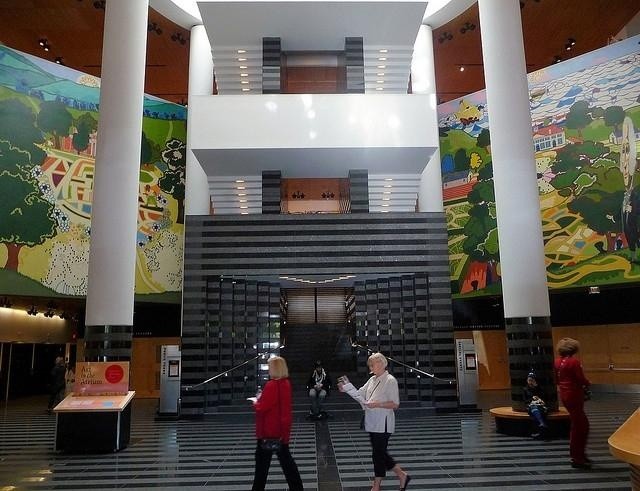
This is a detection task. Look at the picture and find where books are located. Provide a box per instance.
[343,383,365,404]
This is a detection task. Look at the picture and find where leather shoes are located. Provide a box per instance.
[400,474,410,491]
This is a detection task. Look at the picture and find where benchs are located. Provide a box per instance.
[488,403,574,441]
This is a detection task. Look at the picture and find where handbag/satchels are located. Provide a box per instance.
[360,410,365,429]
[530,396,545,406]
[261,438,281,452]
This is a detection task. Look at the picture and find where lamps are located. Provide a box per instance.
[551,38,577,66]
[291,190,305,200]
[0,296,73,321]
[93,0,187,45]
[321,192,336,199]
[38,37,63,66]
[437,21,475,43]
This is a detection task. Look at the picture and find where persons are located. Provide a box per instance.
[306,359,329,418]
[523,371,550,438]
[337,352,411,491]
[555,337,594,468]
[45,356,66,415]
[246,356,304,490]
